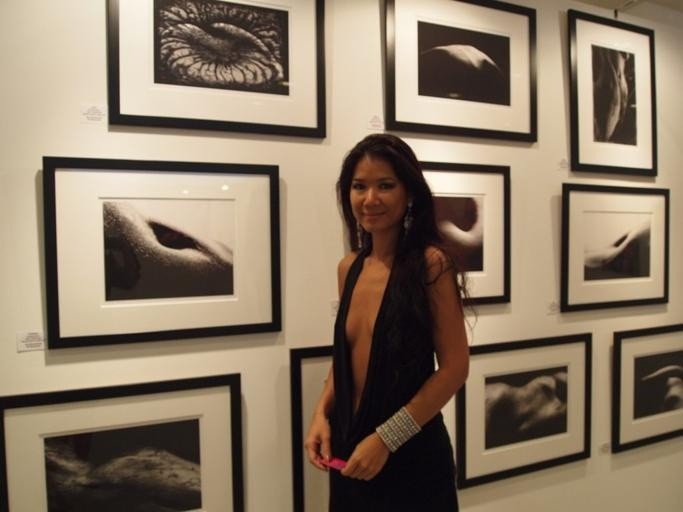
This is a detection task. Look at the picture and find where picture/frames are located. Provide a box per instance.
[105,0,326,139]
[612,323,683,454]
[561,183,669,312]
[419,161,511,304]
[0,373,244,511]
[567,8,658,176]
[290,345,334,512]
[456,333,592,489]
[43,155,281,348]
[385,1,537,142]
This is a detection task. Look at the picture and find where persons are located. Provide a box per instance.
[304,135,478,512]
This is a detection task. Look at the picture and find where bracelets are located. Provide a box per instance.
[374,406,420,454]
